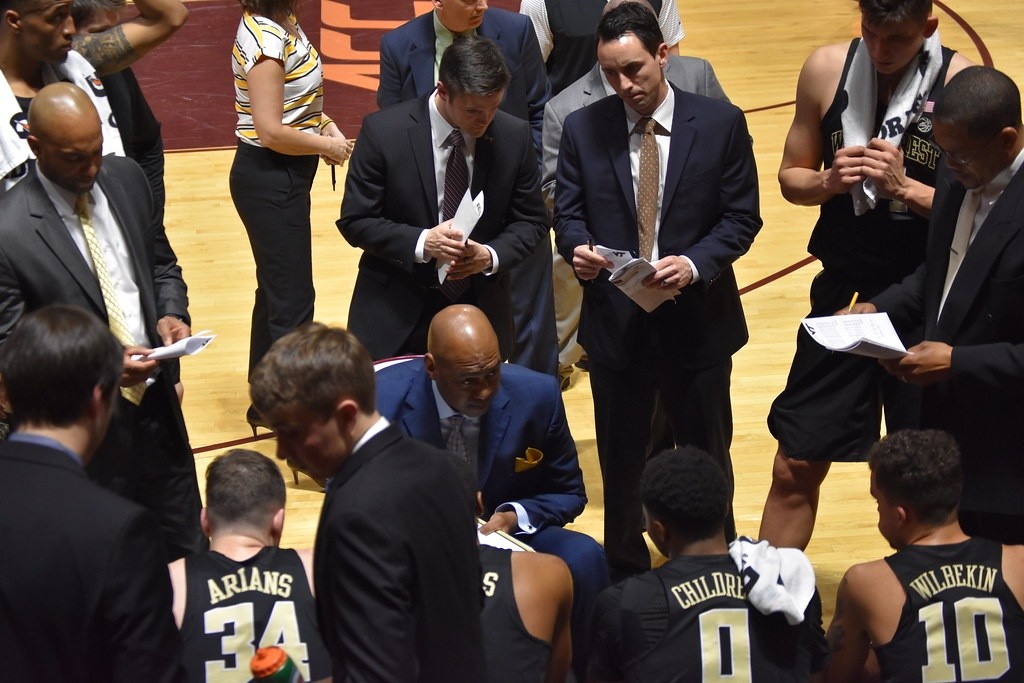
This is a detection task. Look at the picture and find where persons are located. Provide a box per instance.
[0,0,1024,682]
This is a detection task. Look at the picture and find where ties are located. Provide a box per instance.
[447,416,472,467]
[440,132,472,303]
[635,118,657,263]
[937,183,985,324]
[452,32,463,43]
[76,189,147,406]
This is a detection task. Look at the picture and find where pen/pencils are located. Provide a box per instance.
[588,234,594,284]
[330,164,336,191]
[831,291,859,355]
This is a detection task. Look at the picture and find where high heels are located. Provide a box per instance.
[246,402,278,438]
[287,455,326,488]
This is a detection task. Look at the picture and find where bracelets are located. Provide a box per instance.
[321,119,334,130]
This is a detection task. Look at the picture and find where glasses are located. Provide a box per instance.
[927,125,1001,169]
